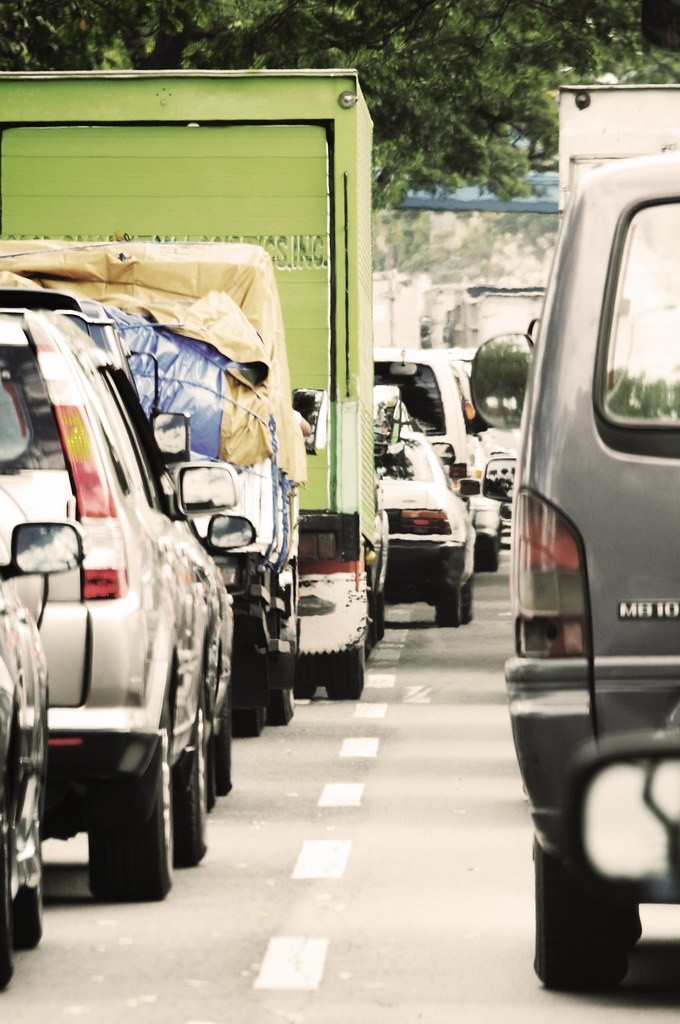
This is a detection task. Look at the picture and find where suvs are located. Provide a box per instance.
[1,307,210,902]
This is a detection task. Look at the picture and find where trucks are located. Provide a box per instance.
[0,68,379,702]
[558,85,680,387]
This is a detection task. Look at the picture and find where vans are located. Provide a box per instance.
[468,148,680,993]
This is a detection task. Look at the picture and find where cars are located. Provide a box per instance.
[1,285,256,811]
[374,347,522,628]
[1,582,49,989]
